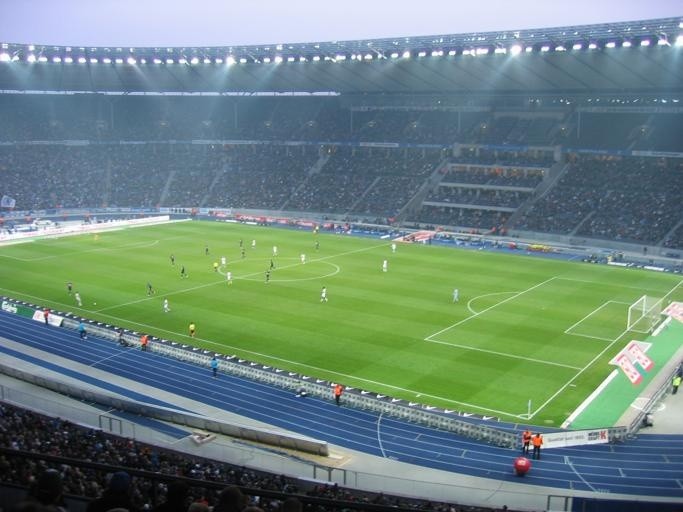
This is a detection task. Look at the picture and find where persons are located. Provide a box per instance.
[335,384,342,404]
[78,322,87,340]
[226,271,232,286]
[521,429,532,455]
[163,298,170,313]
[180,264,187,279]
[451,287,458,303]
[114,329,122,347]
[1,403,350,511]
[382,260,387,272]
[269,259,274,270]
[531,432,543,460]
[319,286,328,303]
[146,282,155,296]
[210,352,218,380]
[345,487,485,512]
[67,282,72,295]
[140,334,148,351]
[265,270,270,284]
[170,254,175,266]
[189,322,195,337]
[0,109,683,264]
[43,309,50,324]
[220,256,225,267]
[213,262,218,272]
[75,291,82,306]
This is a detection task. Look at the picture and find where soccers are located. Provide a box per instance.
[94,303,96,305]
